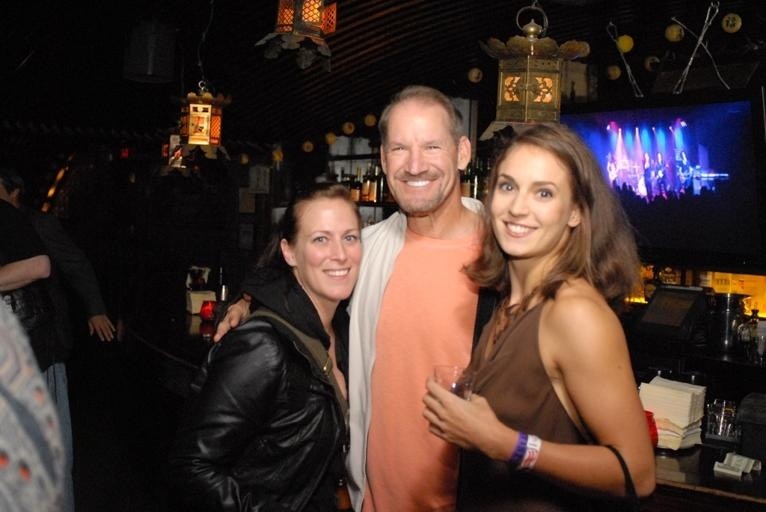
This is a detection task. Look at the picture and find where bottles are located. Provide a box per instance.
[736,308,766,368]
[214,285,230,329]
[460,156,492,201]
[337,161,388,204]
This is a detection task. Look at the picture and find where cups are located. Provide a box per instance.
[432,364,477,401]
[200,301,218,321]
[704,398,738,437]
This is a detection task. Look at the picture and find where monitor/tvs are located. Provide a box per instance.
[561,85,766,277]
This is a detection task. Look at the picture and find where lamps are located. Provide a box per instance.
[173,48,226,152]
[274,0,341,53]
[482,1,592,132]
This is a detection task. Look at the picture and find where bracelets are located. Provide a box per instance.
[508,432,541,477]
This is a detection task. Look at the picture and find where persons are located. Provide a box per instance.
[641,151,654,202]
[418,118,659,512]
[0,164,116,512]
[181,179,367,511]
[654,150,669,196]
[210,81,510,512]
[675,148,694,196]
